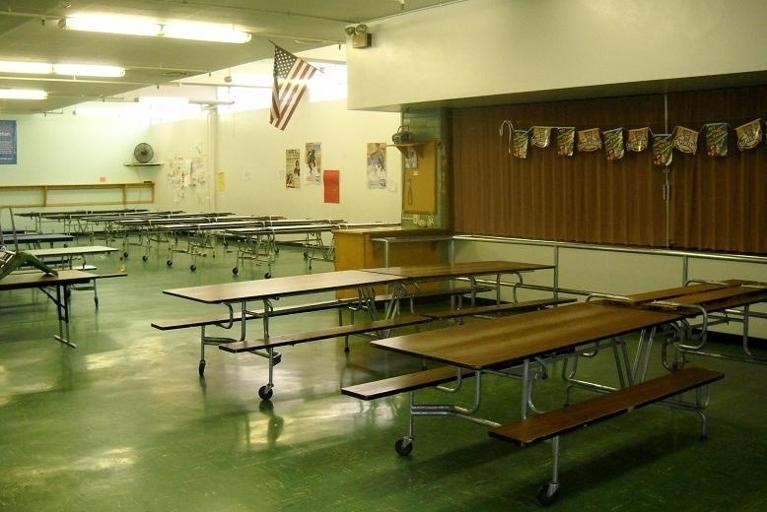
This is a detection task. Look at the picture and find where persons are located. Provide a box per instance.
[304,150,320,178]
[293,156,300,176]
[287,171,293,187]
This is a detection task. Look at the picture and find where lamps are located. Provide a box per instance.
[52,14,251,45]
[345,22,372,49]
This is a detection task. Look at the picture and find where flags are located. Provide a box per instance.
[265,43,318,131]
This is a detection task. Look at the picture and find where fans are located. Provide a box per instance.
[133,142,154,163]
